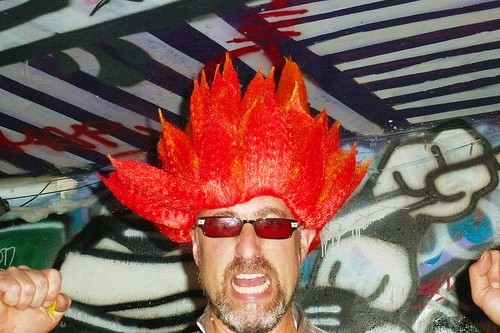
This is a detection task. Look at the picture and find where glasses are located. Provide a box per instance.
[196,216,300,239]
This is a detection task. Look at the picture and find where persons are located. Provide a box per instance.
[0,186,500,333]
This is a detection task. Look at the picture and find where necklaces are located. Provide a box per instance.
[210,307,217,333]
